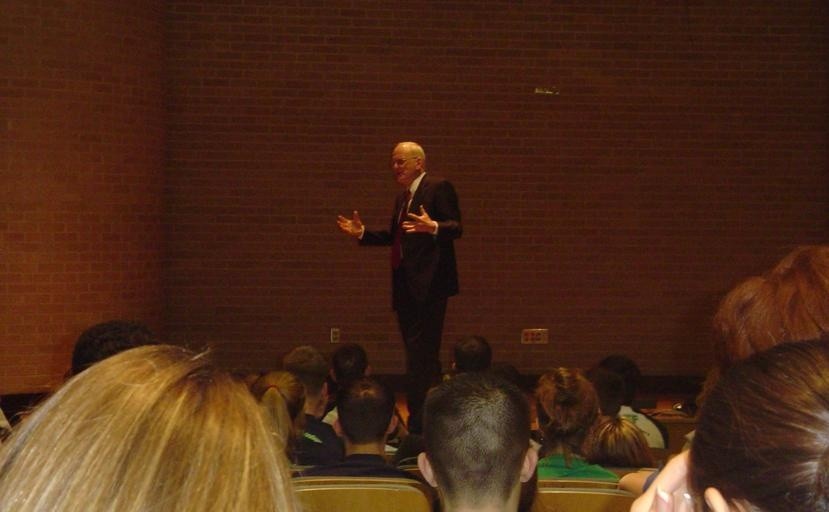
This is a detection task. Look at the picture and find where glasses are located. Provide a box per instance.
[391,156,417,167]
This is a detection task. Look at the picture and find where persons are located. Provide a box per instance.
[0,344,303,512]
[62,321,158,382]
[233,336,670,512]
[629,339,829,512]
[695,245,829,423]
[337,140,464,434]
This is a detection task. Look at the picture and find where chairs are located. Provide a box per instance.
[281,406,703,510]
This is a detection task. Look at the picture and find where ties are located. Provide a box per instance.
[391,189,412,270]
